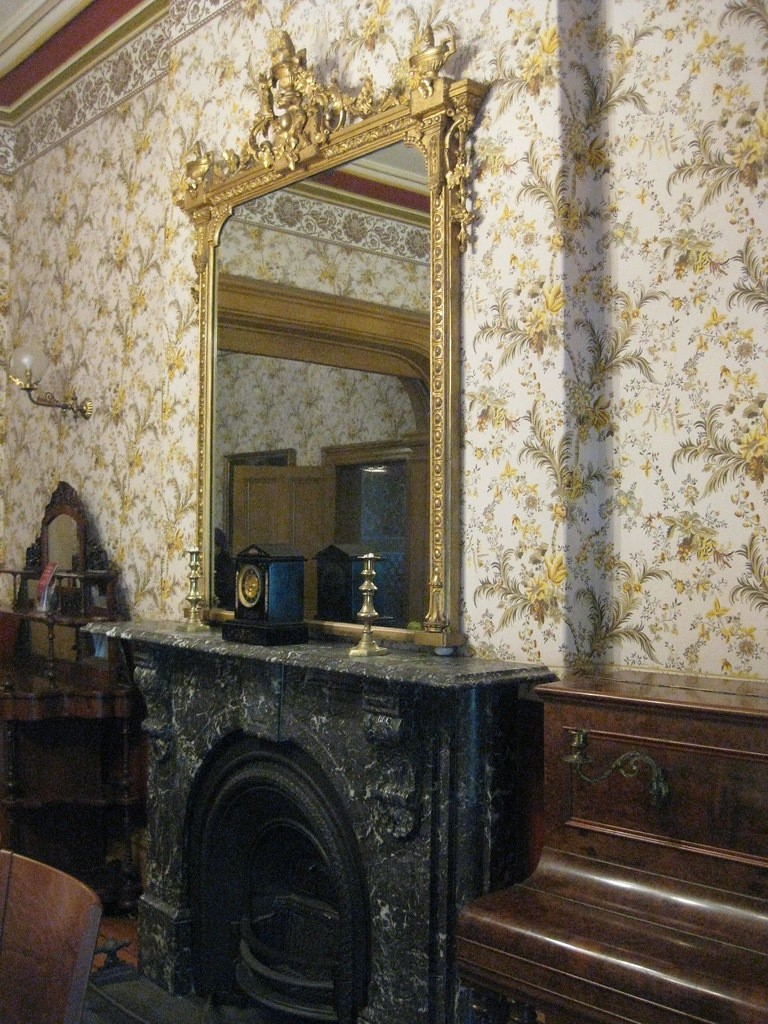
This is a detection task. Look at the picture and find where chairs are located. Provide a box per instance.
[0,849,104,1024]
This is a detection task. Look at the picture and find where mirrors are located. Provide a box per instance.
[169,25,497,652]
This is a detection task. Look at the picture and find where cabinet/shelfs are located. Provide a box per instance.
[2,483,141,918]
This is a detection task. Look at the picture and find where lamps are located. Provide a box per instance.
[6,346,94,420]
